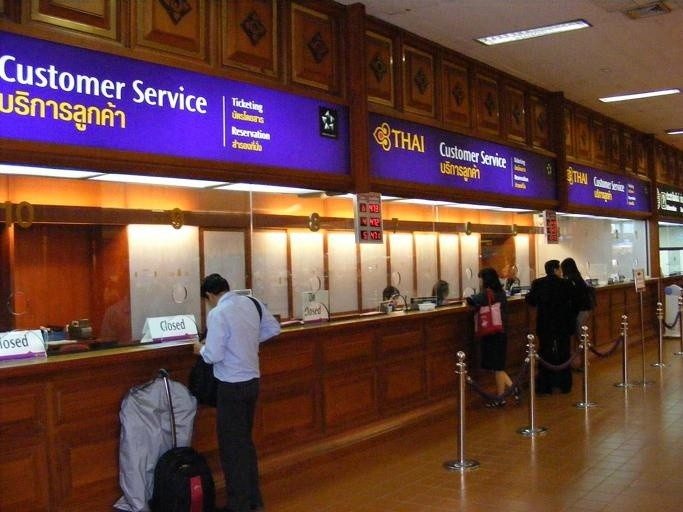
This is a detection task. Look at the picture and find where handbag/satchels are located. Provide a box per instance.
[575,285,597,311]
[471,288,504,337]
[190,359,218,406]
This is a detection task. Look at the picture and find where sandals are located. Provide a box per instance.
[484,397,507,408]
[510,381,521,408]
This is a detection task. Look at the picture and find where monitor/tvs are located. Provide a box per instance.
[410,296,438,309]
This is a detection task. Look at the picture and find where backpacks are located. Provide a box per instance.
[148,446,216,512]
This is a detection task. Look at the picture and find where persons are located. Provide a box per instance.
[504,276,520,292]
[464,266,523,409]
[560,257,594,372]
[524,259,581,395]
[430,279,450,300]
[191,270,283,511]
[381,286,400,302]
